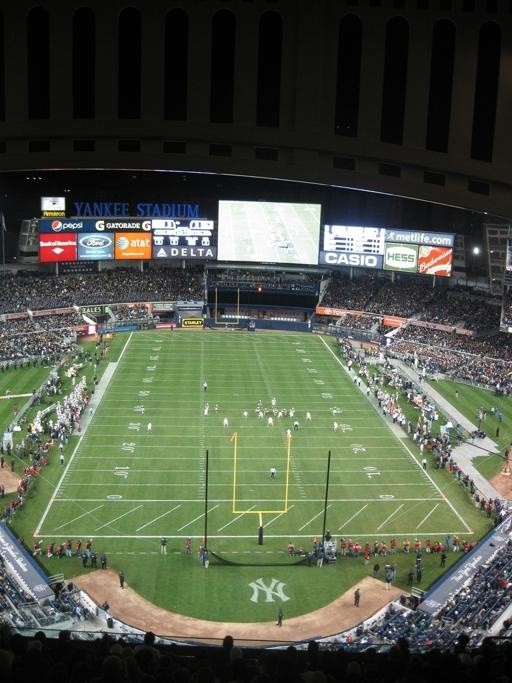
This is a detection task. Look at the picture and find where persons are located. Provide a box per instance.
[0,262,512,683]
